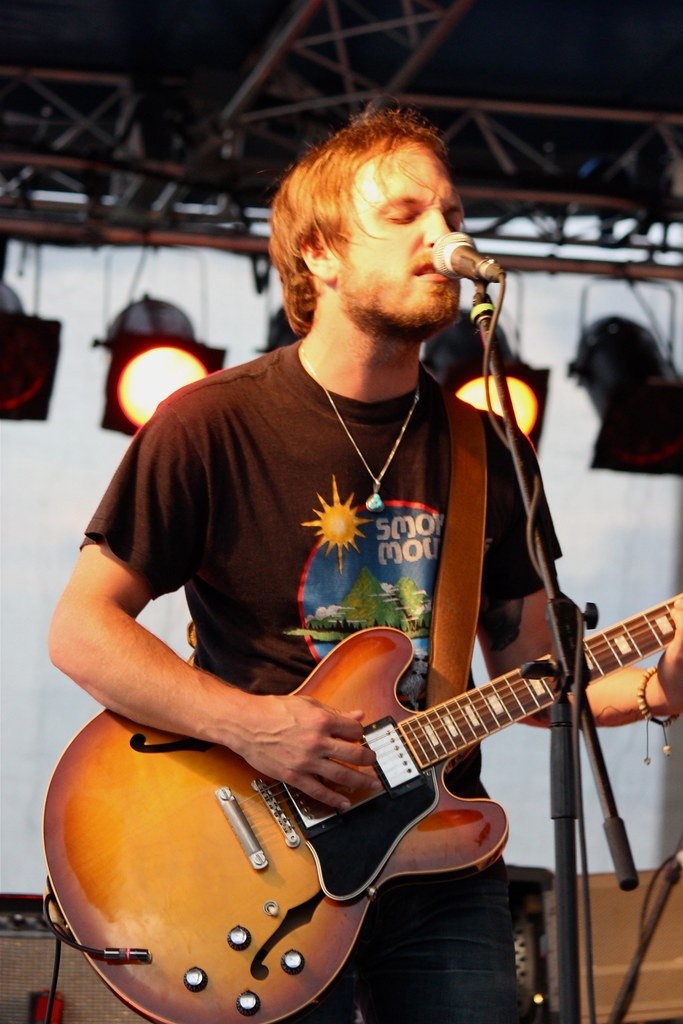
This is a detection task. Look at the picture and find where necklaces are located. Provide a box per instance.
[298,346,422,512]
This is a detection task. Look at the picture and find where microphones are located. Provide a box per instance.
[41,897,151,963]
[432,232,507,284]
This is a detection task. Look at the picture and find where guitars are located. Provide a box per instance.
[39,590,683,1022]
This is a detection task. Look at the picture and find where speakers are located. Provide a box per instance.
[0,893,152,1024]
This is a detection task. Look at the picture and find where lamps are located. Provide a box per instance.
[567,313,683,480]
[424,305,551,451]
[0,264,63,425]
[97,295,228,442]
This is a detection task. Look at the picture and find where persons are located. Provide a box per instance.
[46,115,683,1023]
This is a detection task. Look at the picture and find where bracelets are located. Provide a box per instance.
[636,665,680,765]
